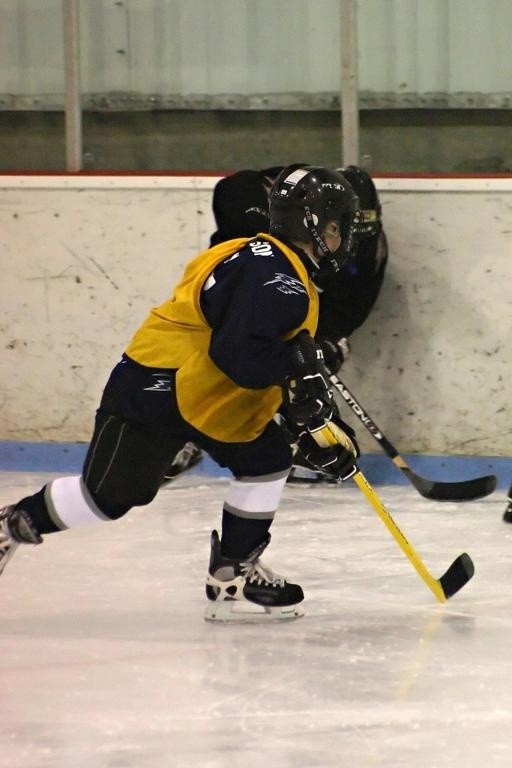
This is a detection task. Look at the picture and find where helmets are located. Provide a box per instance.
[267,164,363,271]
[337,165,382,240]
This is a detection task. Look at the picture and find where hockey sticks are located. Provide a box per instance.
[285,375,476,604]
[320,360,497,502]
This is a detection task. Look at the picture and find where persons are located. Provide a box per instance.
[211,151,388,487]
[0,159,364,610]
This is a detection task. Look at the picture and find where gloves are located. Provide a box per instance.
[288,420,361,479]
[275,365,335,423]
[320,338,350,371]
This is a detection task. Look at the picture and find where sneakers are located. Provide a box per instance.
[165,443,203,477]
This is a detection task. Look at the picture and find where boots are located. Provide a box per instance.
[207,531,303,605]
[0,505,43,574]
